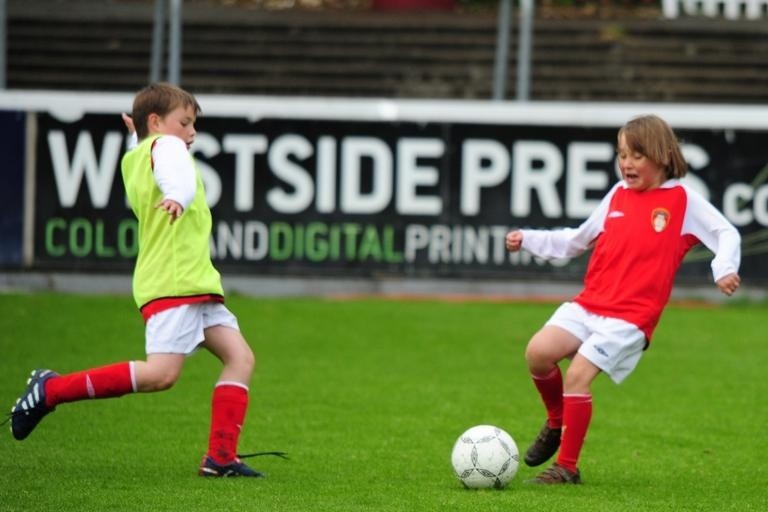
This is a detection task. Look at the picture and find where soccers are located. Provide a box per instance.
[452,424,519,490]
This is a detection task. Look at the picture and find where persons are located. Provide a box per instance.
[9,81,265,478]
[500,114,744,485]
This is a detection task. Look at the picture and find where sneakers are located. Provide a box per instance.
[2,368,61,440]
[199,453,261,479]
[536,465,581,486]
[523,420,560,466]
